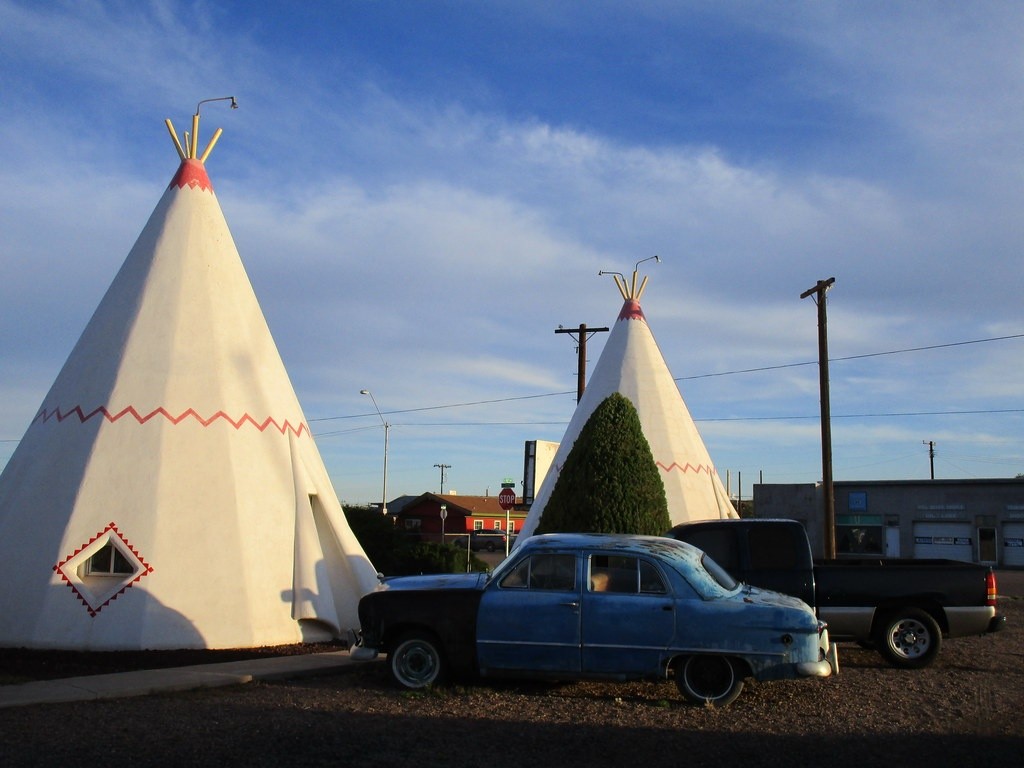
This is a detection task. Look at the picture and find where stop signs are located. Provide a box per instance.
[498,488,516,510]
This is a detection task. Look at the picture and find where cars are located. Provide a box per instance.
[348,533,840,709]
[454,529,518,552]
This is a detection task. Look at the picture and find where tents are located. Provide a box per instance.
[0,157,382,652]
[509,299,741,554]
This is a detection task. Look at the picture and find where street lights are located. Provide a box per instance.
[360,389,392,515]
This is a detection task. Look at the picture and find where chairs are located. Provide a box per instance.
[534,571,573,591]
[590,570,625,592]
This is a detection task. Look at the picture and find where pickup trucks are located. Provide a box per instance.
[659,518,1006,670]
